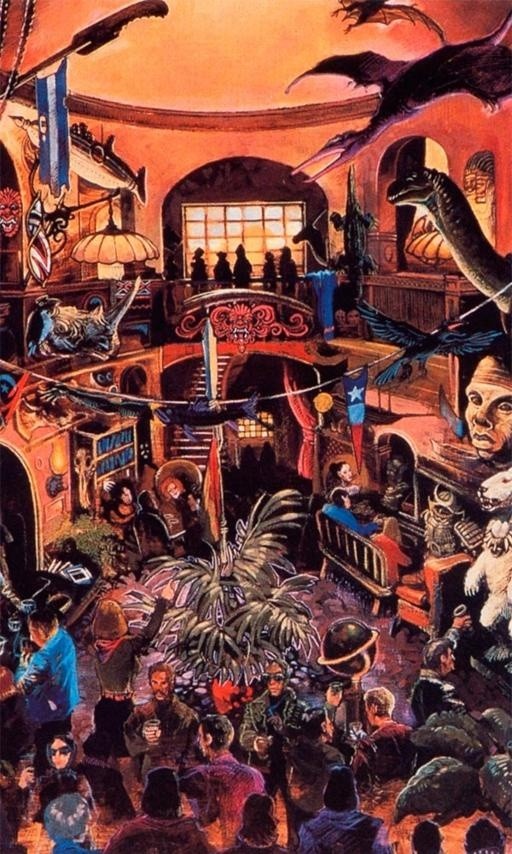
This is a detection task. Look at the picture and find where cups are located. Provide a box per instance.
[145,718,162,747]
[349,720,363,742]
